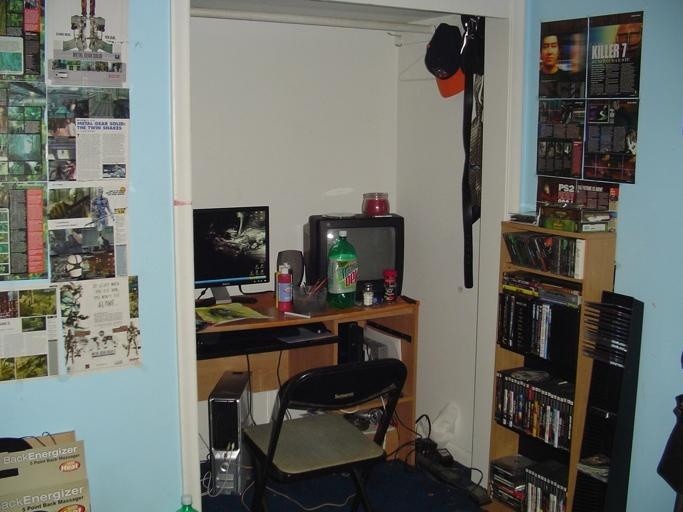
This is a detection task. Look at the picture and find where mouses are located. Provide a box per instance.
[307,319,325,335]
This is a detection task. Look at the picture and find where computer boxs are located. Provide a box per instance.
[209,370,250,494]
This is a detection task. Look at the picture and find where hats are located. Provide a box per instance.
[424,22,464,99]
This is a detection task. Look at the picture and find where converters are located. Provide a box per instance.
[437,448,455,465]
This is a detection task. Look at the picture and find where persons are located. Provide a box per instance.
[539,33,569,98]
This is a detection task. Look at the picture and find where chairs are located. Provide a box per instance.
[243,359,409,512]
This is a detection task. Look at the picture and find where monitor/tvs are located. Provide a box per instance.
[309,212,405,296]
[195,206,269,305]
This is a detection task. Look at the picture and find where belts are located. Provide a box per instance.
[464,17,473,288]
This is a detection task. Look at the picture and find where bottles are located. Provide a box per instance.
[362,283,374,308]
[362,192,391,217]
[325,230,360,310]
[383,268,399,304]
[277,269,294,312]
[275,265,283,309]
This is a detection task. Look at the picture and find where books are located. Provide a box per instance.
[576,453,610,483]
[503,231,585,280]
[498,290,552,360]
[494,365,573,453]
[490,455,539,512]
[525,463,567,512]
[536,202,611,233]
[502,272,582,310]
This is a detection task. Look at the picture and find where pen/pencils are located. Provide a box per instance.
[303,276,330,295]
[285,311,311,318]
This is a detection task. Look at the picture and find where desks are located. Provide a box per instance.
[196,287,421,467]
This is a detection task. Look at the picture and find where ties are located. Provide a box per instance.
[468,73,484,222]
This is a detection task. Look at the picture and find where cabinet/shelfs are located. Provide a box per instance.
[336,309,417,468]
[487,221,615,511]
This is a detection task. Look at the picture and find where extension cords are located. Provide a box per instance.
[414,439,470,483]
